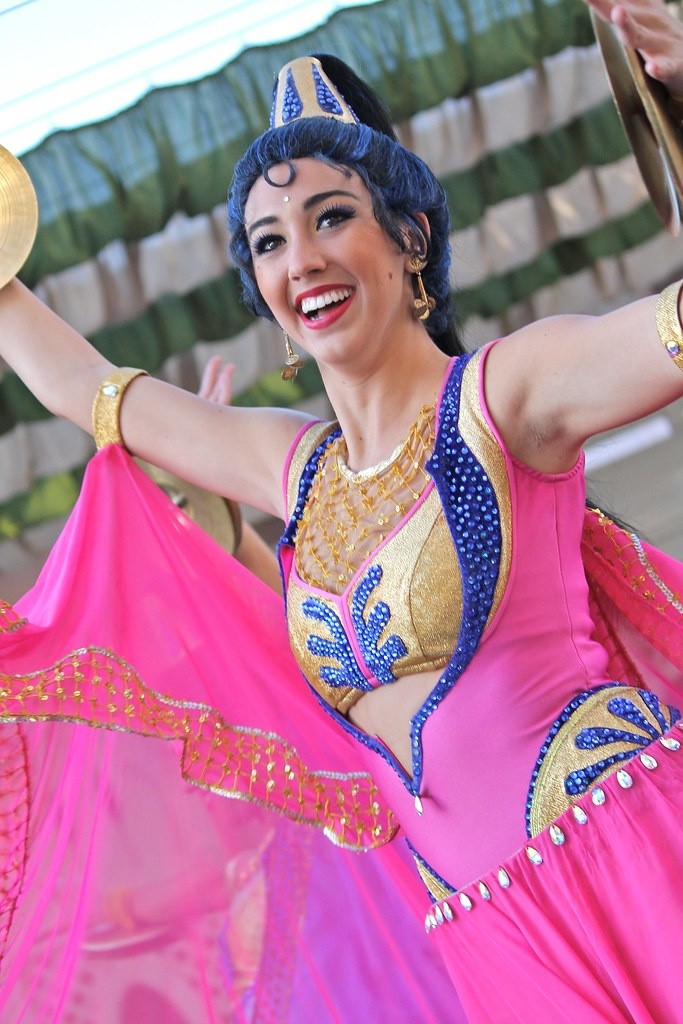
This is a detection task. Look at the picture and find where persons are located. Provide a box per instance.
[0,0,683,1024]
[196,356,283,601]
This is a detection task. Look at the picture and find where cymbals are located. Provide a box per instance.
[588,3,683,237]
[130,455,243,558]
[0,144,39,290]
